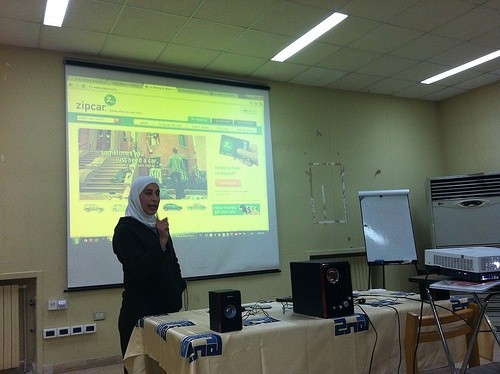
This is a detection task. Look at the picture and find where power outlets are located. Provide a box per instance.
[58,300,68,309]
[92,311,107,321]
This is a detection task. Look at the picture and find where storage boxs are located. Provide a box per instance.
[424,246,500,282]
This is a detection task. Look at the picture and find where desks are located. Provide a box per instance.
[408,274,500,374]
[122,292,494,374]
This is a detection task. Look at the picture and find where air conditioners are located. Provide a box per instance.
[424,171,500,249]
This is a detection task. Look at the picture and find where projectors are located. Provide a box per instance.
[425,246,500,281]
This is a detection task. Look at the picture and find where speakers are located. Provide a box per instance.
[290,260,354,319]
[209,288,242,333]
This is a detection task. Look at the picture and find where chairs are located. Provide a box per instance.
[405,303,481,374]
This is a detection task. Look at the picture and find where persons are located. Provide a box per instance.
[233,143,258,166]
[159,147,187,199]
[112,175,186,374]
